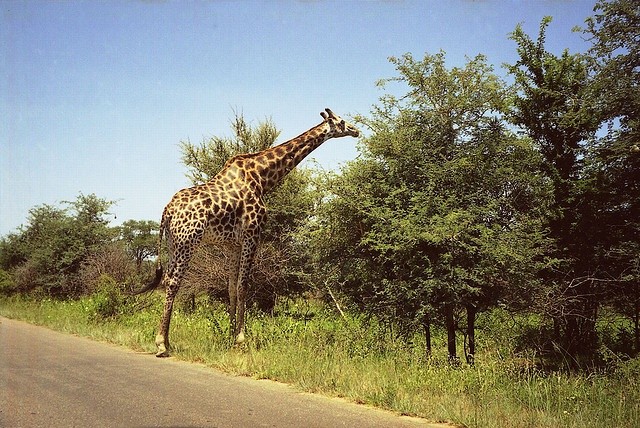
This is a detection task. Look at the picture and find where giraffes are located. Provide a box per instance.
[130,108,359,357]
